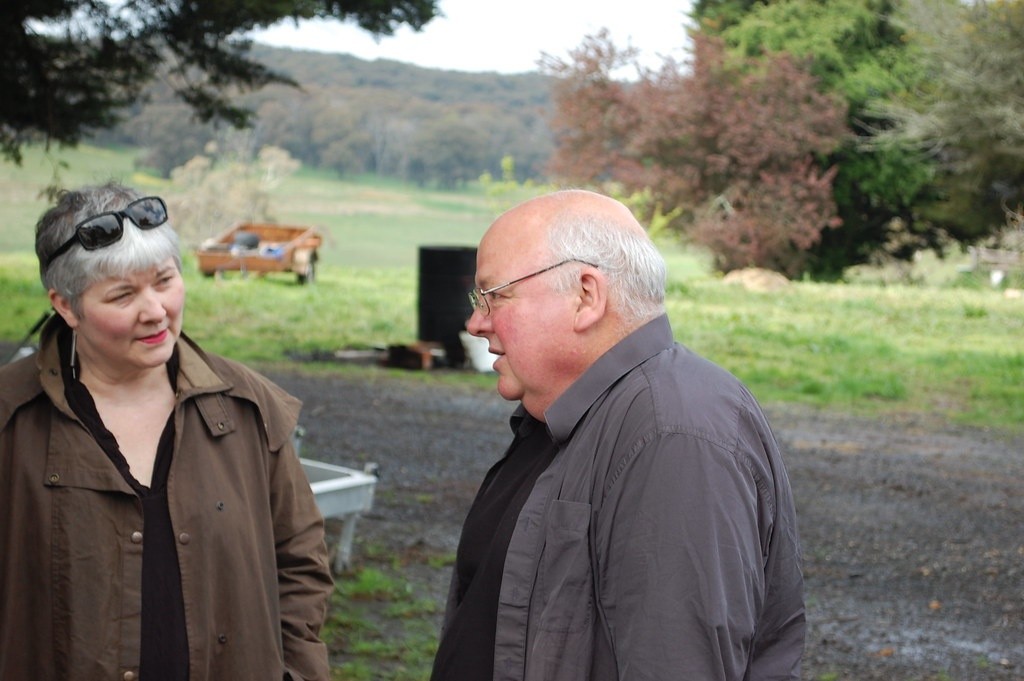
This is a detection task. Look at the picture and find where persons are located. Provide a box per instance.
[427,186,809,681]
[0,186,336,681]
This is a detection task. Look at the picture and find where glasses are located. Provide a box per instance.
[45,195,169,267]
[466,259,597,318]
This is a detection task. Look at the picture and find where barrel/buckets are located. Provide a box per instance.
[417,241,481,365]
